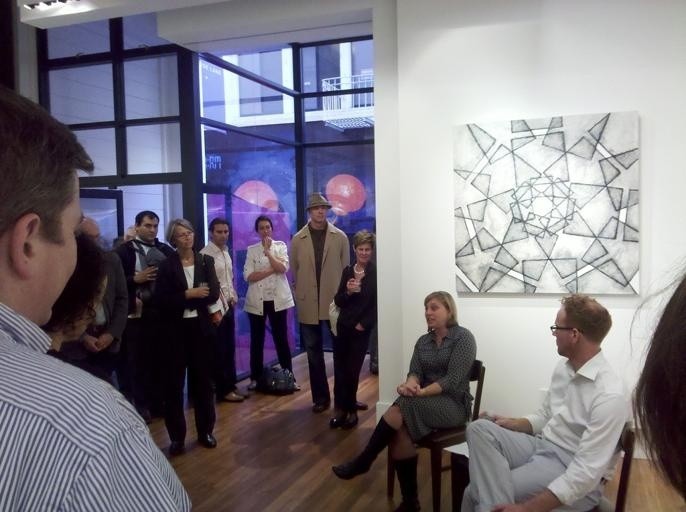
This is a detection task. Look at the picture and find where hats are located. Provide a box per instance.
[305,192,333,211]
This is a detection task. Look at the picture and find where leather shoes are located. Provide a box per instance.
[216,379,300,402]
[196,428,218,449]
[311,400,369,429]
[169,436,186,457]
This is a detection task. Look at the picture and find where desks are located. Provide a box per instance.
[447,444,471,511]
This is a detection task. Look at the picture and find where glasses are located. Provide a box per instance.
[174,232,193,240]
[549,324,573,333]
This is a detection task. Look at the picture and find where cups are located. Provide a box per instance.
[351,279,360,292]
[199,282,209,297]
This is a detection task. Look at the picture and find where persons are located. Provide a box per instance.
[2,85,195,511]
[47,189,377,456]
[633,270,686,506]
[332,290,478,512]
[460,292,633,511]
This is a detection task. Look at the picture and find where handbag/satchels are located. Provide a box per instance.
[328,297,341,336]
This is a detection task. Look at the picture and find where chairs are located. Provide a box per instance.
[613,420,635,512]
[382,360,487,512]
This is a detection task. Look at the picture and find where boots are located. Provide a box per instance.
[331,414,399,480]
[392,453,422,511]
